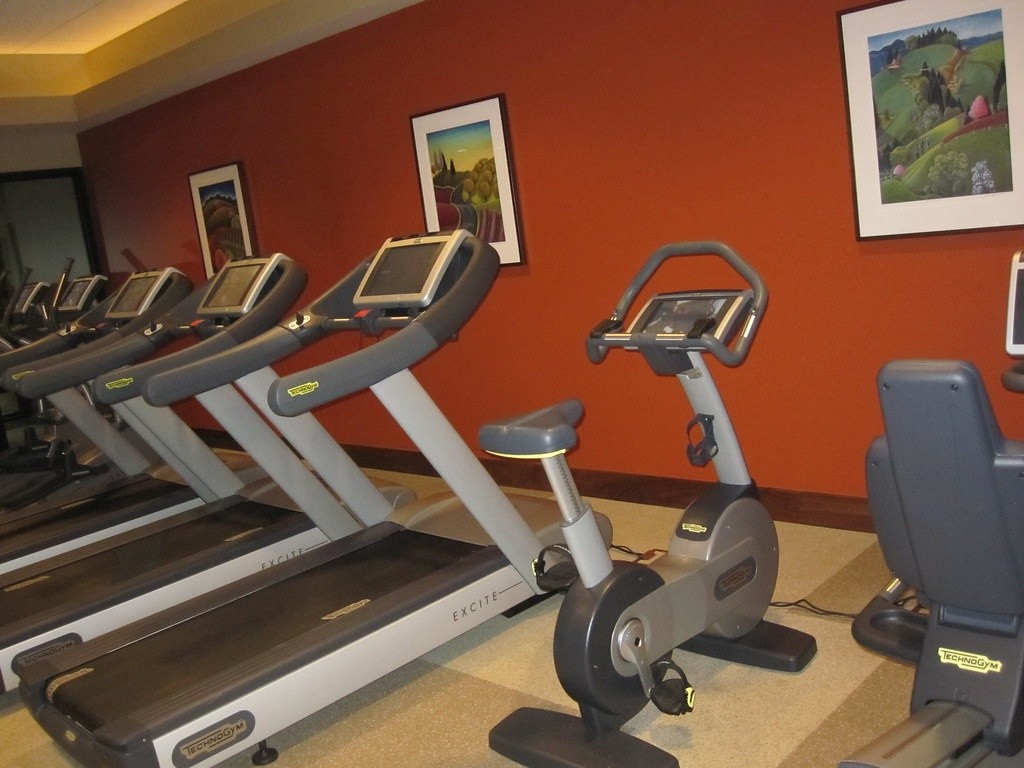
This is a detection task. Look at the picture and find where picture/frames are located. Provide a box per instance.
[835,0,1024,241]
[410,93,526,268]
[188,162,258,282]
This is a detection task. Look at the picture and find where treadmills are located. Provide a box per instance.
[0,227,615,767]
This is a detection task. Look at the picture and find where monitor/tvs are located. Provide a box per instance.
[58,280,92,306]
[110,275,159,313]
[203,263,266,308]
[15,287,34,310]
[360,240,447,298]
[630,295,738,335]
